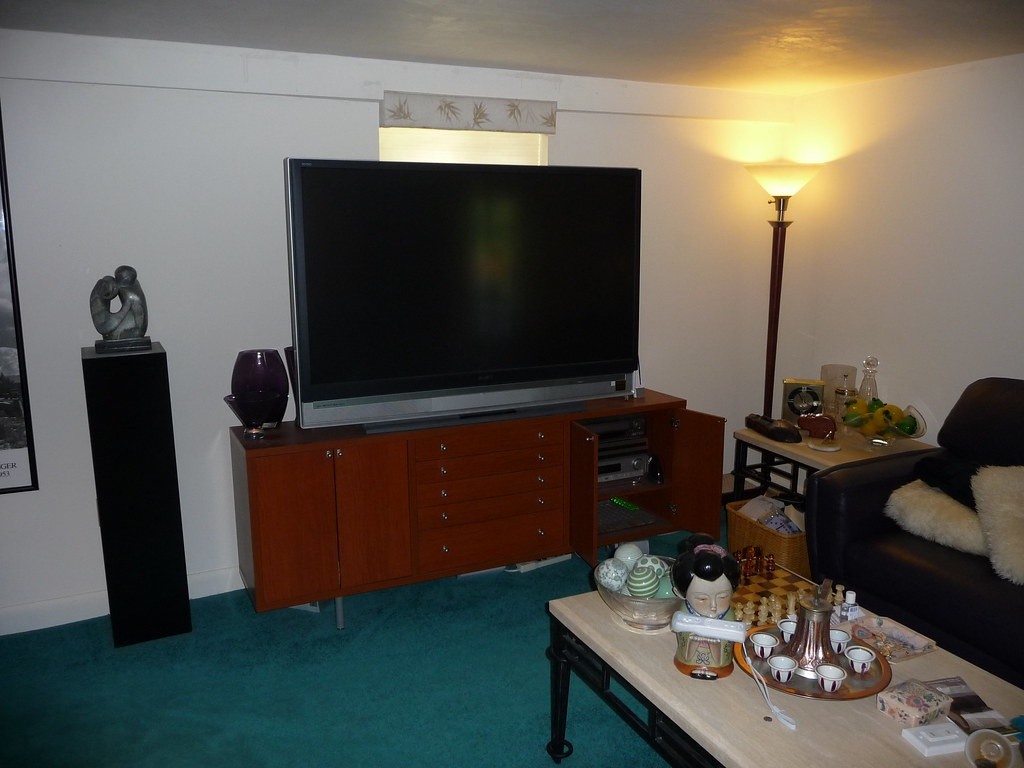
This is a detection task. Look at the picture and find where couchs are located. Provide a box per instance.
[804,378,1024,688]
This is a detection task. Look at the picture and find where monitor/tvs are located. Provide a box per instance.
[283,157,639,437]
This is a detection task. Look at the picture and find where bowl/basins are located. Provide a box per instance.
[594,544,682,630]
[767,654,799,683]
[224,392,285,440]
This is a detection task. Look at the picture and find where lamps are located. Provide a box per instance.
[746,162,819,481]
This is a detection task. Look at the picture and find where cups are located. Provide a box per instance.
[845,643,876,673]
[814,663,847,693]
[778,672,785,679]
[749,632,780,658]
[829,628,852,653]
[776,618,797,642]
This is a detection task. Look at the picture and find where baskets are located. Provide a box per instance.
[727,498,810,580]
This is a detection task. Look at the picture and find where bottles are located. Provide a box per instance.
[840,591,857,622]
[231,349,289,427]
[834,387,856,424]
[858,356,878,404]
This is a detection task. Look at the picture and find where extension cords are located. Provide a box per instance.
[514,553,572,573]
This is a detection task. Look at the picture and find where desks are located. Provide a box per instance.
[544,557,1024,767]
[731,425,936,517]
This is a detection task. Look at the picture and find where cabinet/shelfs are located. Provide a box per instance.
[228,386,728,630]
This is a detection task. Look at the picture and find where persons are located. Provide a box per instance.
[670,534,747,681]
